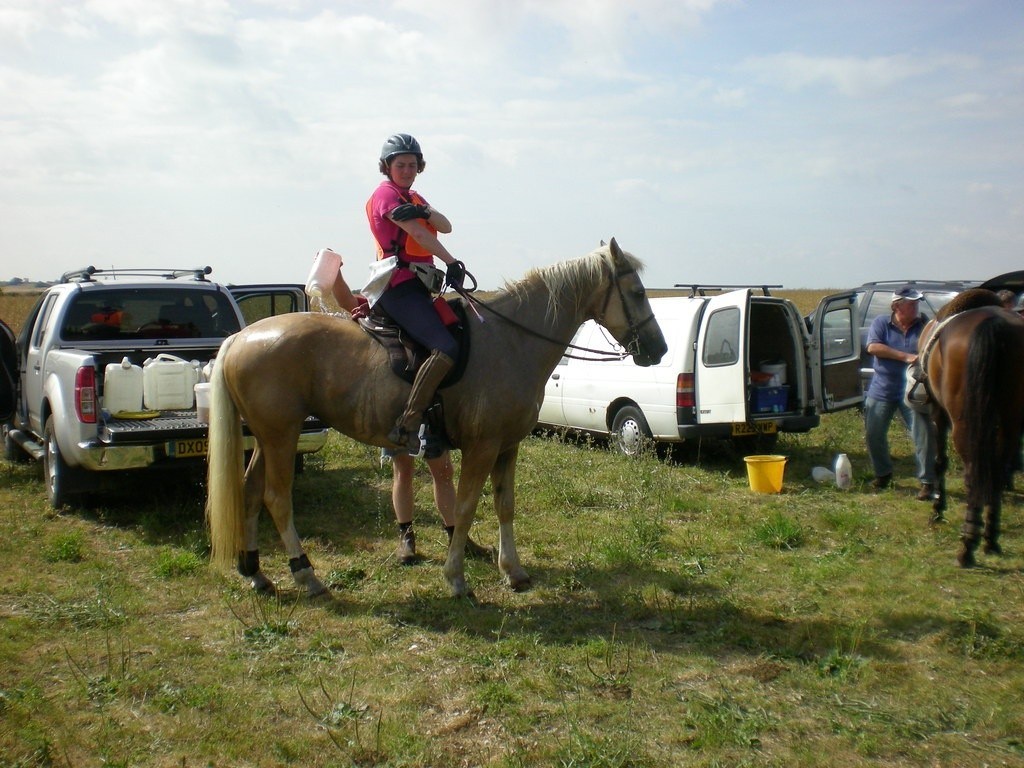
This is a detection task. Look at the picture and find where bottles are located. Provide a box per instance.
[304,248,342,306]
[836,453,853,489]
[101,408,111,421]
[812,466,835,483]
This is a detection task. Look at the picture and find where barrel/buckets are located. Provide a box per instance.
[902,362,931,416]
[761,358,787,385]
[142,353,193,411]
[744,454,789,495]
[103,356,143,414]
[189,358,216,400]
[193,383,212,423]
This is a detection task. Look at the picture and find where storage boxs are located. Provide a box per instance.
[754,385,794,413]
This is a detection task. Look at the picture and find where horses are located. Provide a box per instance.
[920,293,1024,568]
[207,237,667,601]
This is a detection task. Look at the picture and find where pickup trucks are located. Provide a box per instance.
[0,266,331,511]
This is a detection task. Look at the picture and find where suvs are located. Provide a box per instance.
[803,270,1024,400]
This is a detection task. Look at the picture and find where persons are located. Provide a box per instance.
[316,248,500,565]
[863,286,935,500]
[366,133,463,455]
[996,290,1024,317]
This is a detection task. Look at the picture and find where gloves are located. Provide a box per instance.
[445,261,461,288]
[391,202,431,222]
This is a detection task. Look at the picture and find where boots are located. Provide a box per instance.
[386,349,454,458]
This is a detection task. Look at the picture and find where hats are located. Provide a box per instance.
[891,284,923,302]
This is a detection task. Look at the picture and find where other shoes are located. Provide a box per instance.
[872,474,892,487]
[918,484,932,500]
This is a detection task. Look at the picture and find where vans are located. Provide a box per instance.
[528,284,869,458]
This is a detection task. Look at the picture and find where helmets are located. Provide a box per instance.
[380,133,422,161]
